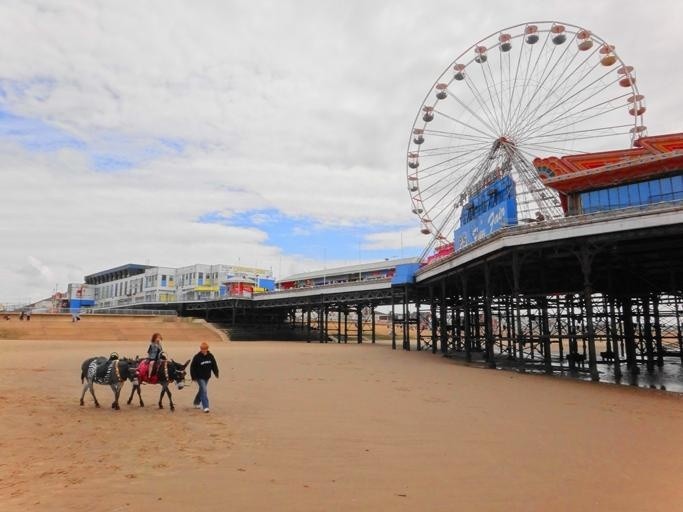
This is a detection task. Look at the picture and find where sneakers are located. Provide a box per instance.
[204,408,210,413]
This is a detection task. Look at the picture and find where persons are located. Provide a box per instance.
[190,343,219,414]
[145,333,165,379]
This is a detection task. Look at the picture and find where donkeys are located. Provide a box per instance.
[80,356,140,410]
[127,358,191,411]
